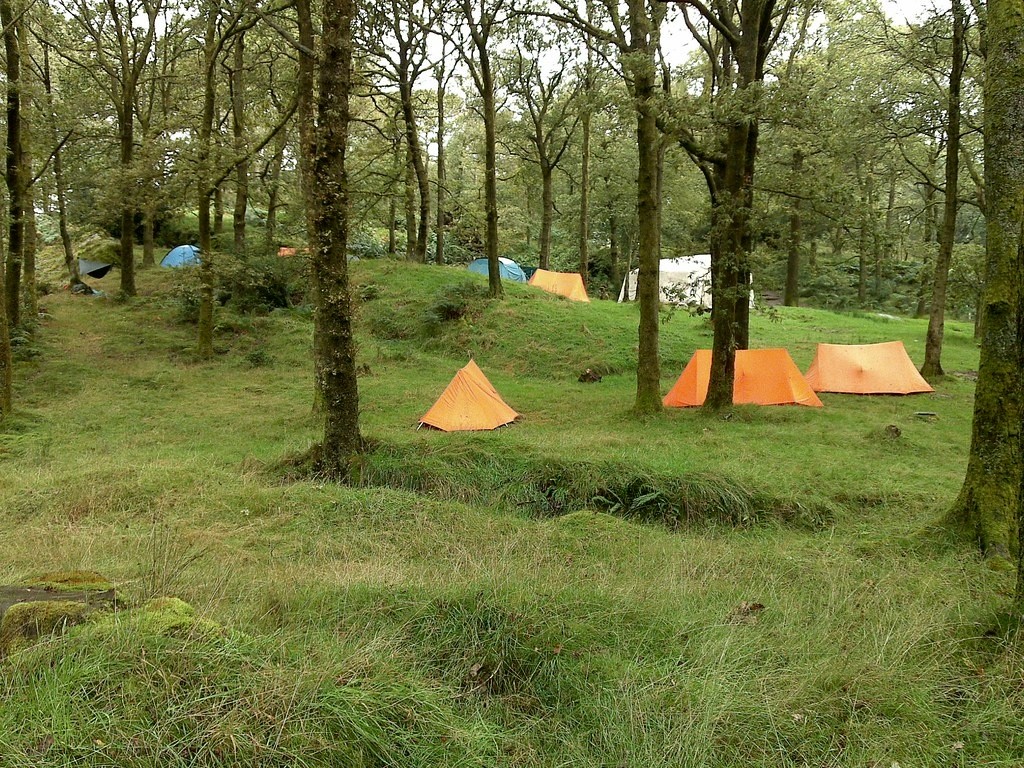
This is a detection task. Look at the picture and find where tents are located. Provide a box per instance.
[660,347,826,410]
[415,357,522,433]
[158,243,202,269]
[802,339,937,396]
[616,253,758,311]
[277,246,310,257]
[467,255,591,304]
